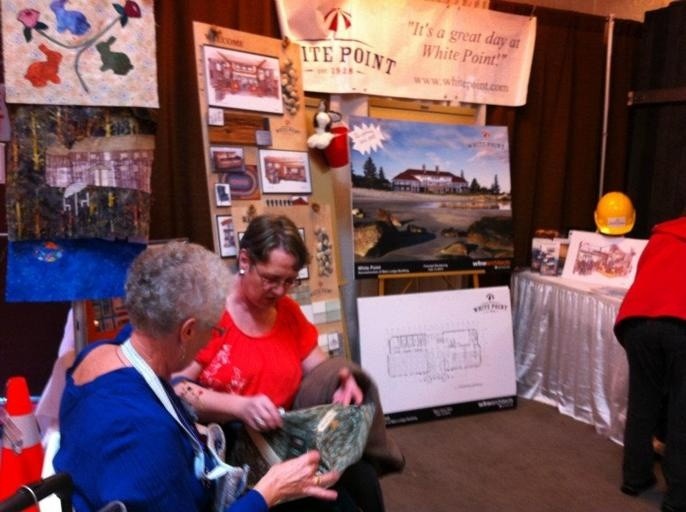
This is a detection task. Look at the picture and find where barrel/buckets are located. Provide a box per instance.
[323,126,350,168]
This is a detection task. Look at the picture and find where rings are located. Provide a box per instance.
[314,475,321,486]
[253,416,265,428]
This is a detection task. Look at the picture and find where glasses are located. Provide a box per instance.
[252,258,301,290]
[210,324,225,338]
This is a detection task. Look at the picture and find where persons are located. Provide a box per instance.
[5,106,152,245]
[50,240,385,512]
[612,214,686,511]
[164,212,365,480]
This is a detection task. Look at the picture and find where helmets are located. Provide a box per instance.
[594,191,637,235]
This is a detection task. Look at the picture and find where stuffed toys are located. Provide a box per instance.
[304,98,346,150]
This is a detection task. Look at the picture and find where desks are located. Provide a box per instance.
[510,269,629,446]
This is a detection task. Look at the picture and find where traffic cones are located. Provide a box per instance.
[0,376,46,512]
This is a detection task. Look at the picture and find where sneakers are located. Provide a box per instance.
[620,475,657,495]
